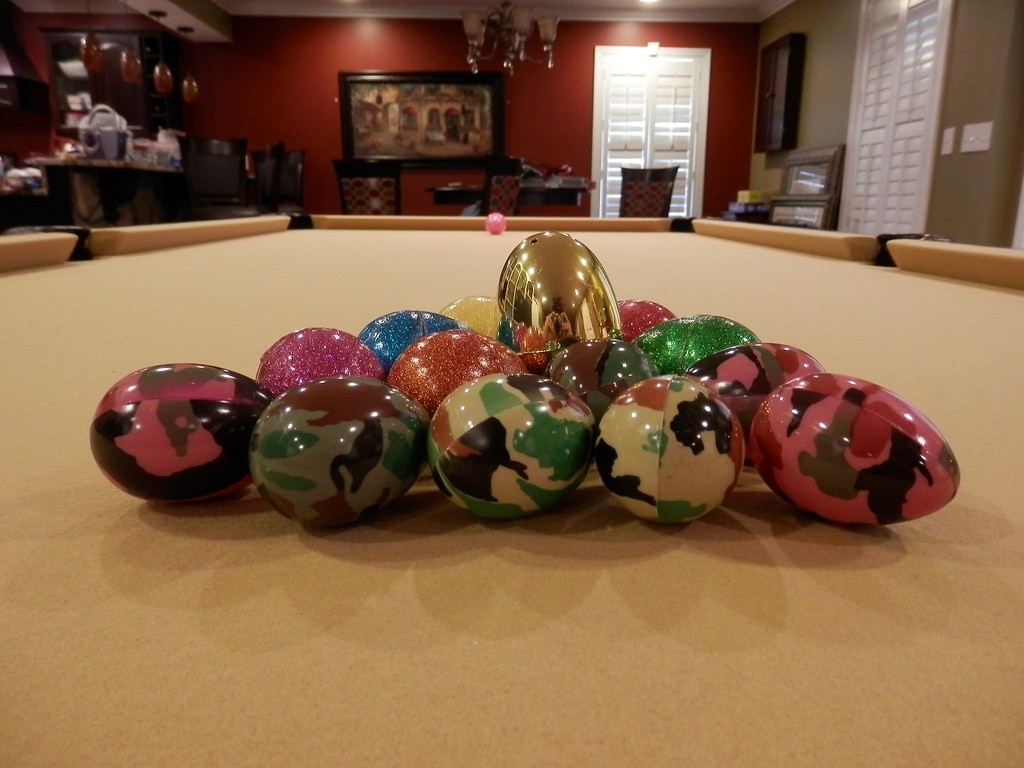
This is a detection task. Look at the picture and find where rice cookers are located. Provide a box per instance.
[79,104,127,161]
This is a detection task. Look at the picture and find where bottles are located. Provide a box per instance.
[158,129,181,166]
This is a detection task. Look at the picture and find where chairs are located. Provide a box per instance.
[182,137,259,221]
[479,156,522,219]
[618,164,680,220]
[247,140,285,215]
[278,149,307,214]
[331,160,401,215]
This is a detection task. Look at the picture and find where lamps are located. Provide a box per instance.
[461,0,559,76]
[118,0,143,84]
[148,10,173,93]
[177,27,200,104]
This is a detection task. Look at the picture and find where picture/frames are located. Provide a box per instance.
[337,71,507,170]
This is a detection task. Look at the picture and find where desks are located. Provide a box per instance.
[27,156,185,227]
[426,170,596,217]
[0,209,1024,768]
